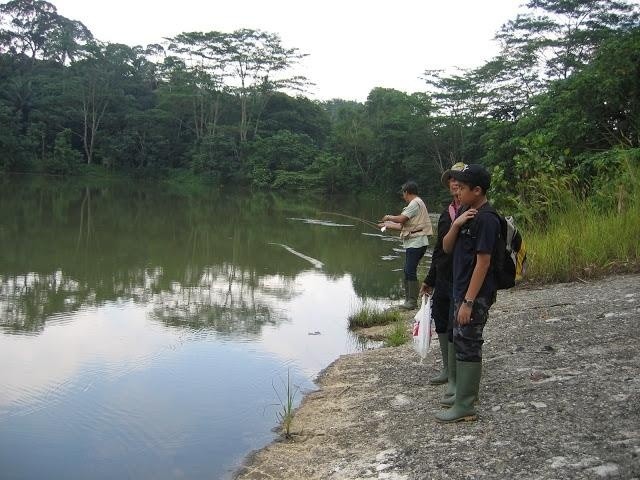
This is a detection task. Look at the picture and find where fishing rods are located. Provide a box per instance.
[319,212,383,230]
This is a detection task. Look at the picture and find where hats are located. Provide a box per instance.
[440,162,464,184]
[450,164,490,187]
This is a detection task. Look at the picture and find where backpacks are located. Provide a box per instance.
[481,208,526,289]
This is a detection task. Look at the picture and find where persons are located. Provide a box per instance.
[432,162,502,424]
[377,181,433,311]
[417,161,475,398]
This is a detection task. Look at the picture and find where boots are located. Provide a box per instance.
[430,333,448,384]
[441,394,457,407]
[401,280,409,306]
[403,280,419,309]
[434,361,483,423]
[445,343,456,396]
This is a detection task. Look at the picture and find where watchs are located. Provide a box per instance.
[462,298,475,308]
